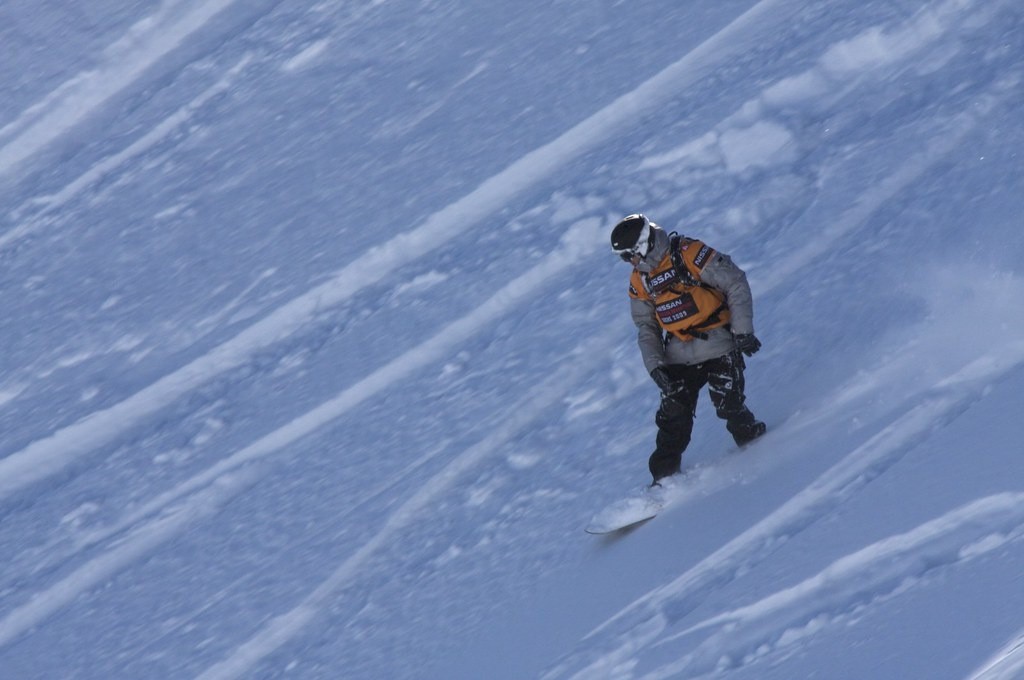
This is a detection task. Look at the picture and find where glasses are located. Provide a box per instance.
[619,253,635,262]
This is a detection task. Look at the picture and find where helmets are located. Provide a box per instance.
[609,213,653,267]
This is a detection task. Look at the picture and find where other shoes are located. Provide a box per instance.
[735,420,765,444]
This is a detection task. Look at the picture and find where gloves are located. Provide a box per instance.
[733,335,763,357]
[650,366,672,395]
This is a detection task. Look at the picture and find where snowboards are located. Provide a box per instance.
[584,452,750,536]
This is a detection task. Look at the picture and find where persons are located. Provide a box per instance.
[611,216,767,492]
